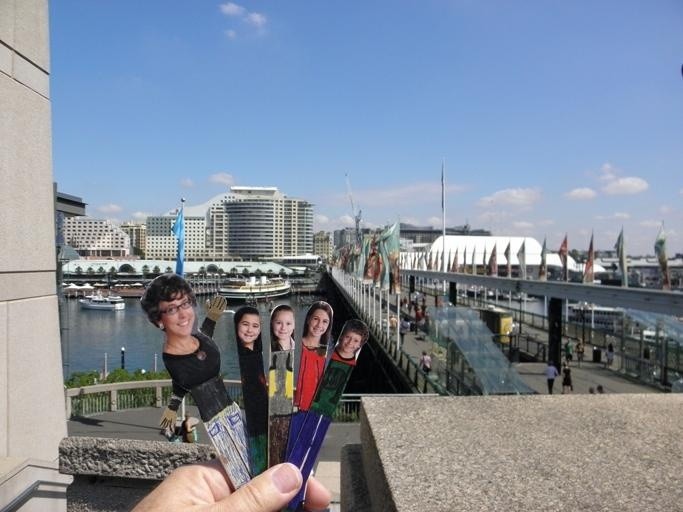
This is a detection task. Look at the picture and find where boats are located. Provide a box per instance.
[78,289,126,312]
[215,276,292,304]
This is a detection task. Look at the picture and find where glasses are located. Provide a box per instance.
[162,297,193,315]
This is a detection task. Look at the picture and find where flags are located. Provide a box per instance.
[170,206,184,279]
[402,219,671,292]
[333,212,401,294]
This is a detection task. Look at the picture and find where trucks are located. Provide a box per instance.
[566,302,625,324]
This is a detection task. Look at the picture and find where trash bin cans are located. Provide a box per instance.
[593,345,601,362]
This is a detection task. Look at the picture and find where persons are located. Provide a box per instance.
[605,341,615,366]
[597,385,607,393]
[563,337,573,367]
[294,300,334,411]
[574,338,585,367]
[176,411,196,444]
[139,272,232,429]
[131,456,333,512]
[311,317,370,418]
[231,303,267,440]
[642,342,651,360]
[417,349,432,384]
[588,386,596,393]
[560,360,572,394]
[380,295,428,346]
[269,302,294,417]
[542,360,558,394]
